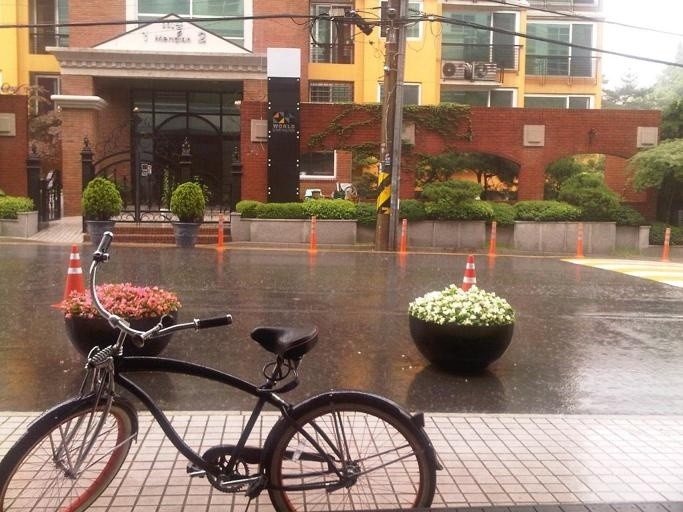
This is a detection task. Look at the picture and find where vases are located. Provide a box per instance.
[409,312,517,372]
[64,311,178,359]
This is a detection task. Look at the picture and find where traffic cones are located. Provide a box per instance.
[459,253,477,292]
[51,242,89,310]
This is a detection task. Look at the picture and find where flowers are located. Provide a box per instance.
[408,283,516,326]
[62,282,182,321]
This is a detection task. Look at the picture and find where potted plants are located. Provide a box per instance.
[81,178,122,246]
[170,182,206,248]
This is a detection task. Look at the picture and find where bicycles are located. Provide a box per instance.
[0,230,443,511]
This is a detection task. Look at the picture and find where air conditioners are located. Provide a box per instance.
[470,61,497,80]
[440,60,465,79]
